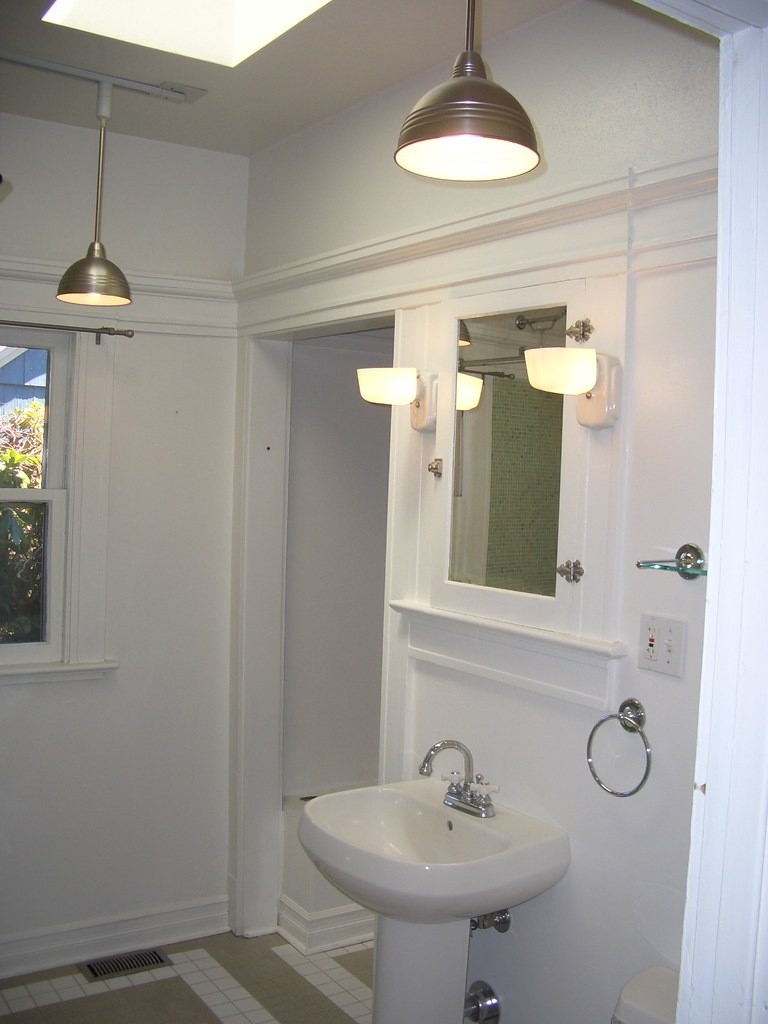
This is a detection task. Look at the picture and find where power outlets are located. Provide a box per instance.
[635,612,688,679]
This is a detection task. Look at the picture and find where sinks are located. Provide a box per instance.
[297,776,571,923]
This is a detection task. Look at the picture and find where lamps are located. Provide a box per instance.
[458,319,470,346]
[356,367,437,433]
[456,373,483,410]
[54,82,132,307]
[393,0,540,182]
[523,347,621,429]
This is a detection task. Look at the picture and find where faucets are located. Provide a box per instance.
[418,740,499,818]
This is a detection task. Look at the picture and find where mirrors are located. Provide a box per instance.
[446,305,569,598]
